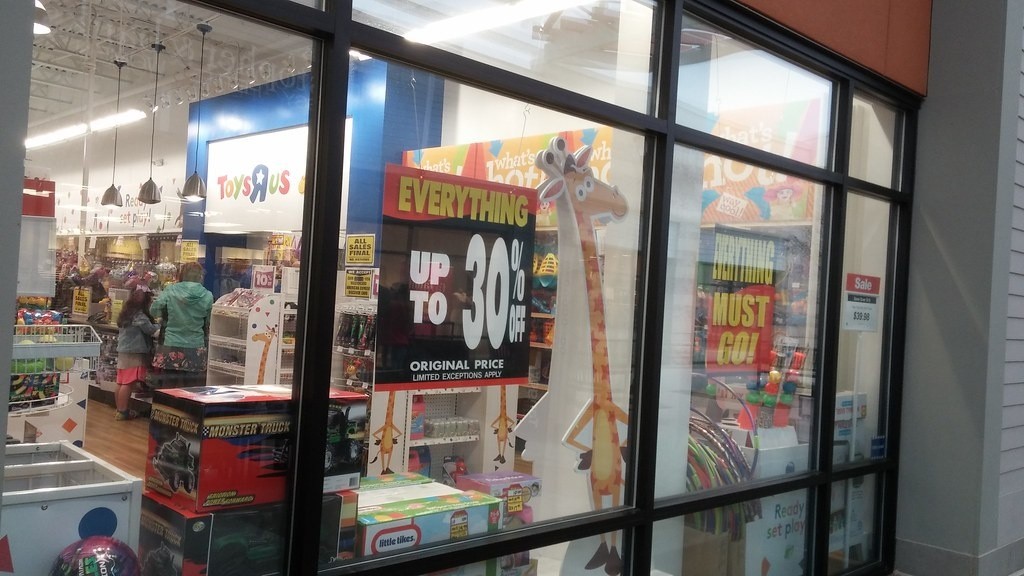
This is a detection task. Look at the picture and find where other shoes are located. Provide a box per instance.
[113,407,142,421]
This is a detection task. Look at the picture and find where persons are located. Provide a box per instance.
[115,289,161,421]
[149,262,214,390]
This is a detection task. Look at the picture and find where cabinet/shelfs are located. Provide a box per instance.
[52,233,300,412]
[330,226,562,475]
[0,438,144,576]
[692,223,813,412]
[7,177,100,450]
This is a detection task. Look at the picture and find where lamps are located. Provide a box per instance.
[182,23,213,202]
[33,0,52,34]
[138,42,165,204]
[102,60,129,206]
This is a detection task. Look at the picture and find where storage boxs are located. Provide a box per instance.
[138,384,545,576]
